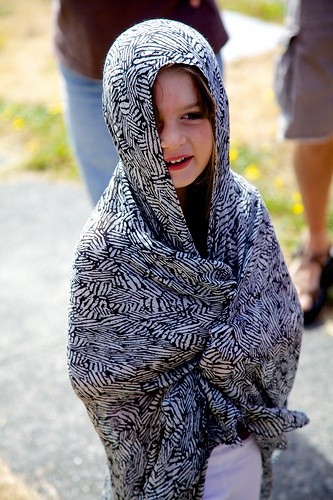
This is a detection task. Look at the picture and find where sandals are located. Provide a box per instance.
[288,249,333,322]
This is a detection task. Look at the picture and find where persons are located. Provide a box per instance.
[271,0,333,332]
[52,0,227,205]
[63,18,312,500]
